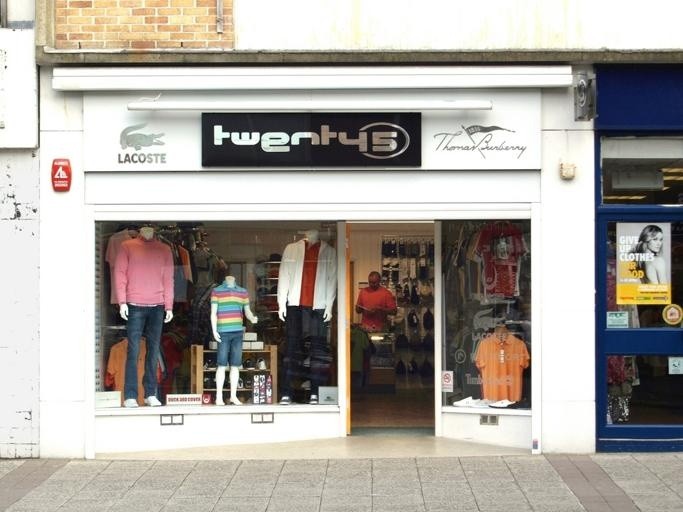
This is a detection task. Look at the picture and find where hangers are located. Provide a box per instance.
[494,220,510,225]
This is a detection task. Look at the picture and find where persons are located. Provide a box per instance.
[355,270,397,332]
[113,227,175,408]
[275,229,339,405]
[208,275,259,406]
[626,224,665,284]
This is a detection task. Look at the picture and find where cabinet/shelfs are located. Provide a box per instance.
[190,345,277,404]
[263,261,281,330]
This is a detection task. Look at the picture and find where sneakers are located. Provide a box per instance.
[308,394,318,404]
[452,394,522,409]
[278,395,292,405]
